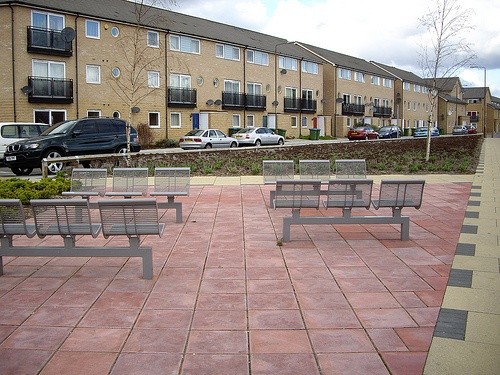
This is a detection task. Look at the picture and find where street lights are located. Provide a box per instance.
[274,40,296,135]
[470,65,486,138]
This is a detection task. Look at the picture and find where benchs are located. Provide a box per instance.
[0,198,166,279]
[267,179,425,242]
[262,159,366,185]
[62,167,190,223]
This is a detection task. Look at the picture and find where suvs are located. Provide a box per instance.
[5,115,141,175]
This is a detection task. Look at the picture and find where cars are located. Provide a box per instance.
[178,129,240,151]
[451,124,477,135]
[231,127,285,148]
[0,120,50,165]
[346,126,380,140]
[413,126,439,138]
[375,125,402,139]
